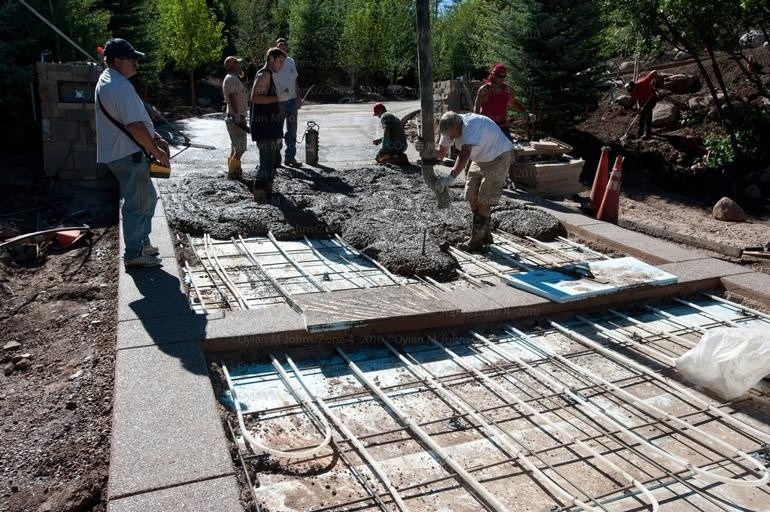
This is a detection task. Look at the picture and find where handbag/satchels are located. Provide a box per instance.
[149,160,171,179]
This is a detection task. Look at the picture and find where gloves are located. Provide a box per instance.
[414,139,425,153]
[234,112,241,124]
[277,94,290,103]
[434,174,455,194]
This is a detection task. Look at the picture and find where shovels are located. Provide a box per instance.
[619,88,656,142]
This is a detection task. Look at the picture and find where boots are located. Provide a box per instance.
[455,213,494,253]
[253,176,276,203]
[227,158,242,180]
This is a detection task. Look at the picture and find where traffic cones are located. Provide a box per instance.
[586,145,612,208]
[595,153,625,227]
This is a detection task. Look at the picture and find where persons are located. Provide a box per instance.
[625,70,657,140]
[94,39,171,267]
[223,38,302,180]
[372,62,531,252]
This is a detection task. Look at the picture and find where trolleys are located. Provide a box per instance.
[498,117,593,208]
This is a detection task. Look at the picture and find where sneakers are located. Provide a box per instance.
[275,159,303,169]
[635,133,652,140]
[124,256,162,269]
[142,245,159,257]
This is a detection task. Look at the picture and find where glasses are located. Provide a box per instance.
[495,75,506,79]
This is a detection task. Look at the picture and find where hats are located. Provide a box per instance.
[372,103,385,117]
[102,38,145,60]
[624,81,635,93]
[224,56,243,71]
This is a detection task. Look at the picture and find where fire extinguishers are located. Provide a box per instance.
[305,120,320,167]
